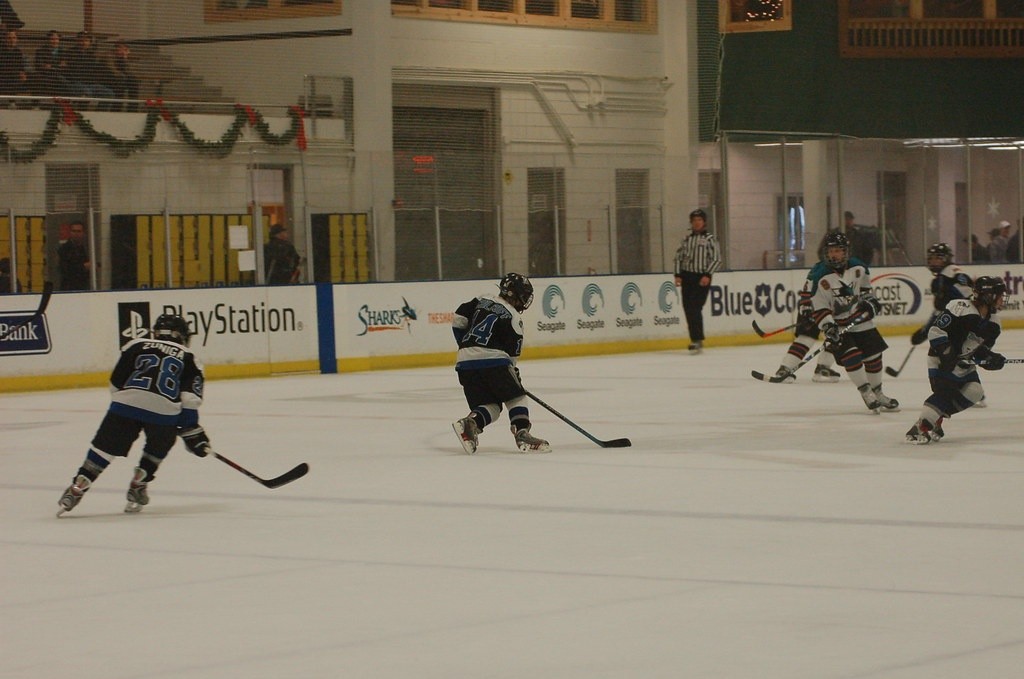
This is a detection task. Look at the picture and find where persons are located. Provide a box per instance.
[776,236,870,383]
[905,275,1006,445]
[0,257,22,296]
[810,233,902,416]
[450,271,552,456]
[57,221,97,291]
[264,224,301,288]
[674,209,722,354]
[832,211,862,260]
[910,243,987,407]
[55,314,211,520]
[964,217,1024,264]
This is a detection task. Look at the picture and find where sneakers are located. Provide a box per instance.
[871,382,900,412]
[688,341,703,355]
[124,467,148,512]
[452,413,483,455]
[929,415,944,441]
[776,365,796,381]
[56,474,91,516]
[510,422,552,452]
[812,364,840,382]
[858,383,881,413]
[905,418,934,444]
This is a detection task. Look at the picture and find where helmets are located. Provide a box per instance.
[690,210,706,223]
[823,233,850,268]
[499,273,533,309]
[926,242,951,272]
[153,314,191,347]
[973,276,1010,310]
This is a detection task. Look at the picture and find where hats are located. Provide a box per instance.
[964,234,977,243]
[845,211,854,219]
[987,229,999,236]
[999,221,1010,228]
[271,224,286,234]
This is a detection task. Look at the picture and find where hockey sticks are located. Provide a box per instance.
[886,346,916,377]
[0,280,53,339]
[752,319,800,338]
[963,359,1023,364]
[752,312,870,384]
[525,390,632,448]
[203,446,309,489]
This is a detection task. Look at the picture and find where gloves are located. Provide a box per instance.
[179,425,211,457]
[822,323,843,352]
[909,322,929,345]
[860,299,881,321]
[972,345,1005,370]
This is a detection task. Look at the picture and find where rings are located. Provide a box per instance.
[705,283,707,285]
